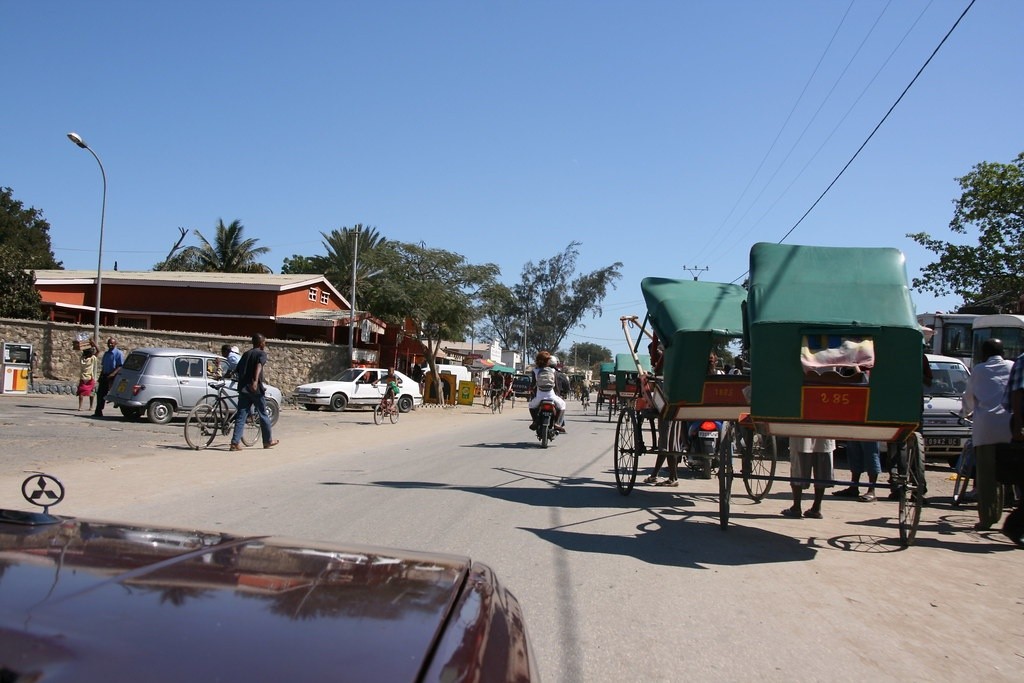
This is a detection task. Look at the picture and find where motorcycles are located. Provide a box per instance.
[534,400,567,449]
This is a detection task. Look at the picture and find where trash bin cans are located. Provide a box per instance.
[458,380,476,406]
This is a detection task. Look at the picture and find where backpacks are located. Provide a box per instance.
[537,367,555,391]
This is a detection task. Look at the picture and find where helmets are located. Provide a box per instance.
[549,356,557,366]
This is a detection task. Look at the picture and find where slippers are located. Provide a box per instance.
[857,495,877,502]
[832,488,860,497]
[803,510,823,519]
[779,509,802,518]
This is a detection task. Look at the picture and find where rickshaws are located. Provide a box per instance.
[595,242,925,548]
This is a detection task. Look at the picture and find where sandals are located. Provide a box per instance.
[644,476,658,486]
[661,479,679,486]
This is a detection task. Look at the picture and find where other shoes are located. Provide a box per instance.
[90,410,103,417]
[264,440,279,448]
[230,444,243,451]
[554,423,566,433]
[529,422,539,430]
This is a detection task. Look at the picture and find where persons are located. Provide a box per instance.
[412,364,426,395]
[489,370,507,405]
[710,352,744,375]
[580,377,592,406]
[528,351,569,434]
[781,352,933,518]
[76,338,124,418]
[207,332,279,452]
[962,339,1024,528]
[371,367,400,415]
[643,340,679,487]
[471,373,480,397]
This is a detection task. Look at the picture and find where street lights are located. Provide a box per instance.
[66,132,107,382]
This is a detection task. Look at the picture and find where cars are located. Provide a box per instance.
[103,347,282,427]
[293,368,424,412]
[509,374,536,402]
[0,473,542,683]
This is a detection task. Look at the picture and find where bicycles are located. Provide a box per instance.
[488,388,505,414]
[184,378,261,450]
[371,384,402,425]
[575,390,588,415]
[949,411,974,506]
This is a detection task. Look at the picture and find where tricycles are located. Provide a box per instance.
[484,364,515,408]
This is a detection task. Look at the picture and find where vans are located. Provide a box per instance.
[923,353,974,468]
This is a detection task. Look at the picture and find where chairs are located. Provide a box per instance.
[180,361,202,376]
